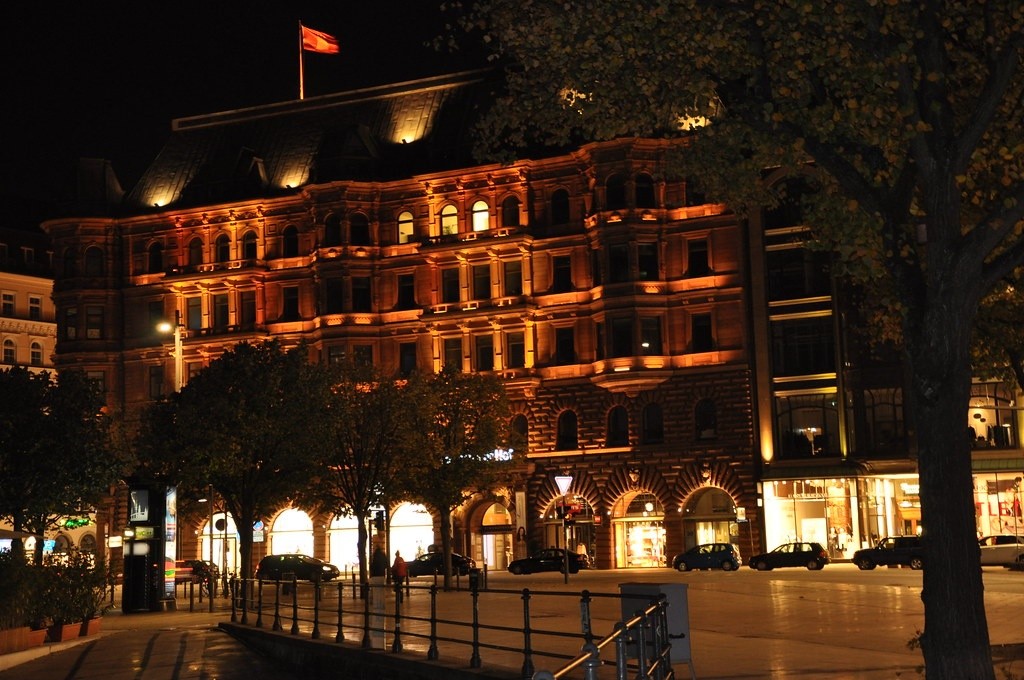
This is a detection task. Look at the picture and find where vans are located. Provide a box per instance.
[255,555,340,582]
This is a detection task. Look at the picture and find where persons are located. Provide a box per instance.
[828,527,848,558]
[391,551,406,591]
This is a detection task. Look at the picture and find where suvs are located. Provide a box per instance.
[851,534,923,570]
[749,542,831,571]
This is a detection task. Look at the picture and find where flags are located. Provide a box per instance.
[302,26,339,54]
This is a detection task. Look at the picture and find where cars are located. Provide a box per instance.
[977,534,1024,566]
[402,553,476,576]
[508,548,587,576]
[673,543,741,571]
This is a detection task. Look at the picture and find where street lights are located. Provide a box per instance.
[156,323,183,394]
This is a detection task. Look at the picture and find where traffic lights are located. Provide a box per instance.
[374,511,384,530]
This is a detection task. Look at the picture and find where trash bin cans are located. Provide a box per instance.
[282,573,295,595]
[618,582,690,666]
[469,567,482,589]
[886,542,898,568]
